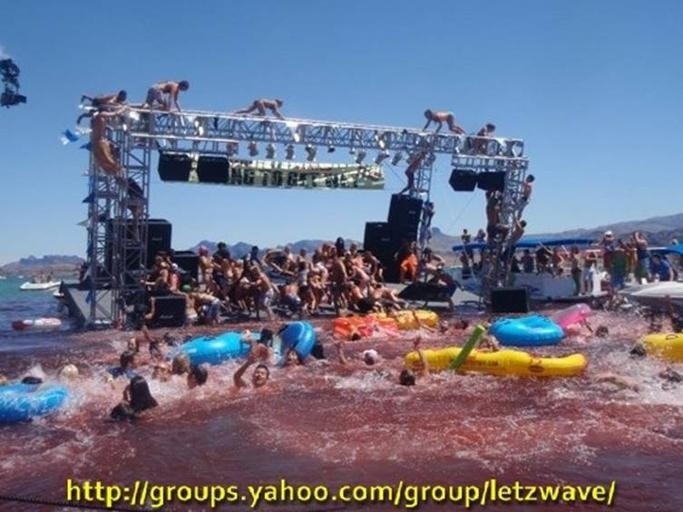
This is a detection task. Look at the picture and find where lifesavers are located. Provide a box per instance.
[0,381,67,426]
[163,332,280,368]
[281,322,315,363]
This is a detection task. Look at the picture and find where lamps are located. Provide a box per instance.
[226,139,403,166]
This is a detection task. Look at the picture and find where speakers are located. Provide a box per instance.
[448,168,476,191]
[364,193,424,282]
[198,156,229,183]
[149,296,186,328]
[492,287,529,313]
[108,219,198,284]
[478,172,505,190]
[157,154,192,181]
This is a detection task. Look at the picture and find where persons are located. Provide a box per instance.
[2,78,683,423]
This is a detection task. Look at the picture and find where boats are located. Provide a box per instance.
[19,280,63,290]
[14,317,59,330]
[0,375,70,423]
[365,307,442,331]
[398,297,683,386]
[170,318,315,368]
